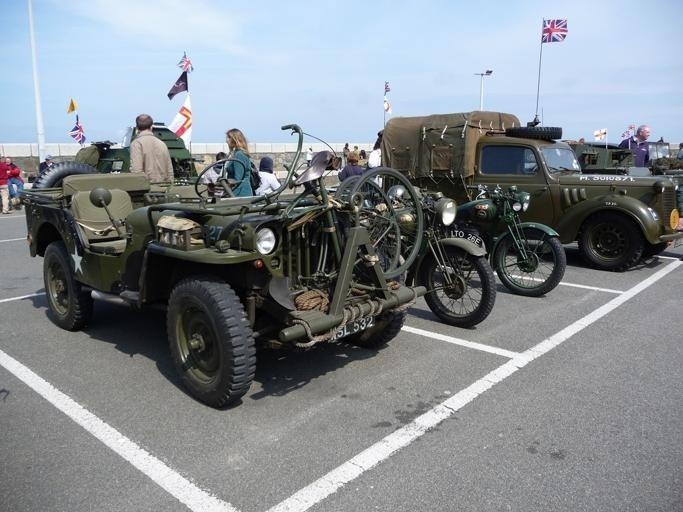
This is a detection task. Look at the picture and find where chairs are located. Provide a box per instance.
[69,190,133,245]
[166,184,209,204]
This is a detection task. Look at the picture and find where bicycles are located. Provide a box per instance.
[250,123,424,280]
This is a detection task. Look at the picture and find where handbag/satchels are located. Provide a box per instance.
[249,161,263,191]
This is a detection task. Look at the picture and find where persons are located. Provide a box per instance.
[202,152,227,184]
[3,157,24,208]
[38,155,53,174]
[222,128,254,197]
[617,125,650,169]
[676,143,682,160]
[251,155,282,196]
[336,135,382,193]
[307,148,312,167]
[129,113,175,195]
[0,154,11,214]
[578,138,584,145]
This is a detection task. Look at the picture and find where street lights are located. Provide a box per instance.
[475,70,492,111]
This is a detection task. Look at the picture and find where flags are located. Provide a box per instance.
[540,20,567,45]
[384,80,391,94]
[383,96,391,114]
[66,115,85,144]
[177,55,193,75]
[164,96,191,151]
[66,98,75,113]
[594,129,606,142]
[166,71,186,102]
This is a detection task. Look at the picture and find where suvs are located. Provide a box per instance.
[18,161,427,407]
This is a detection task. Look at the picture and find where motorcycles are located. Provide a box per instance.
[381,184,566,326]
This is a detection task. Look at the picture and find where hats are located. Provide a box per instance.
[44,155,52,160]
